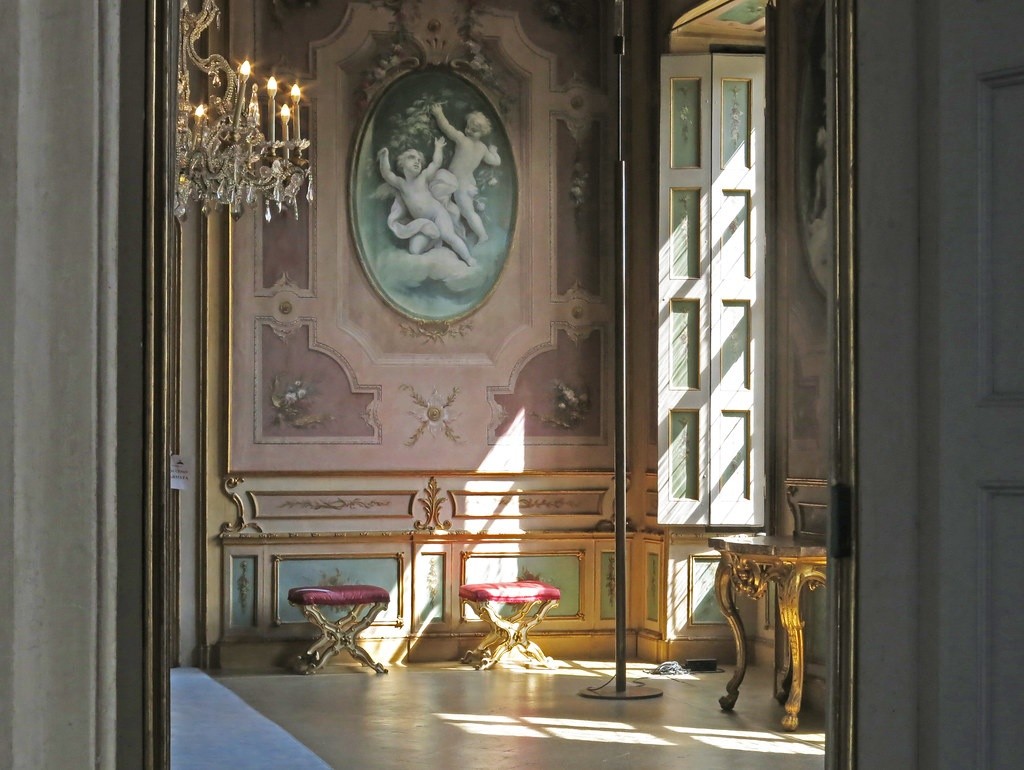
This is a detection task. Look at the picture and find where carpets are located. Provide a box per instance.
[171,667,334,770]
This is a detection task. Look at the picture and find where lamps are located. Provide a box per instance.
[174,0,314,221]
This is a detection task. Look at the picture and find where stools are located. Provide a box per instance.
[458,581,561,670]
[287,585,390,675]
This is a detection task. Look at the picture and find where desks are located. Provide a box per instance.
[707,535,827,731]
